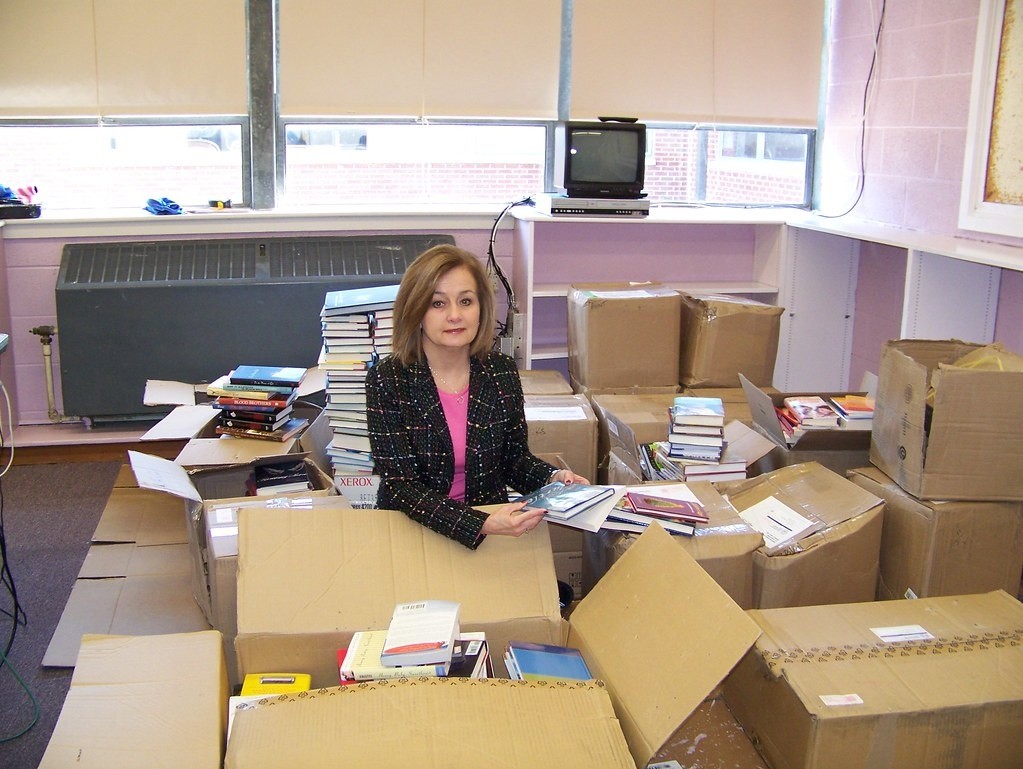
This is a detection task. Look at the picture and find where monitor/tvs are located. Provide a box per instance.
[562,120,646,200]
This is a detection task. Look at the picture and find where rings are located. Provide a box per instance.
[526,528,528,534]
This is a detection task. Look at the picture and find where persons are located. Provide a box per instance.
[366,243,590,551]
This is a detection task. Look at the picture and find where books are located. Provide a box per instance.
[774,394,874,449]
[511,480,710,538]
[227,599,593,747]
[319,285,402,476]
[206,365,310,442]
[244,460,314,497]
[637,397,748,482]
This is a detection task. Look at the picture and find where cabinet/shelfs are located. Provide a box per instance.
[513,206,787,398]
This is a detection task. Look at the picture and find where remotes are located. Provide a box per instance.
[598,116,639,123]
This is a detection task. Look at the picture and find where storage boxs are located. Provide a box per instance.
[36,280,1023,769]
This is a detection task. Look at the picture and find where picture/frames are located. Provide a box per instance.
[958,0,1023,237]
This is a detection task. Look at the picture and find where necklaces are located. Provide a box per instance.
[429,360,469,404]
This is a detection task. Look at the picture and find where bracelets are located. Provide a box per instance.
[547,469,562,485]
